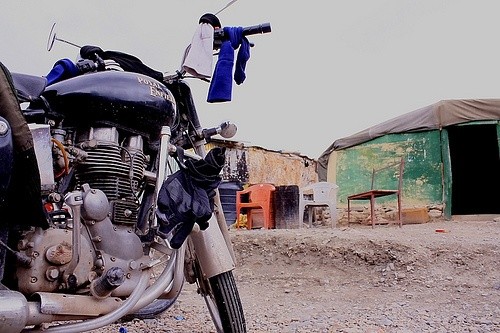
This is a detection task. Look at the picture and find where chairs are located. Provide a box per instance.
[346,156,405,229]
[235,181,277,230]
[299,180,341,228]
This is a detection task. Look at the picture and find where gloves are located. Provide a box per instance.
[157,146,226,249]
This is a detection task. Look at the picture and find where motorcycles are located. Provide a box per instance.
[0,0,272,333]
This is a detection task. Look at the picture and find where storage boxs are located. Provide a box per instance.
[394,205,429,225]
